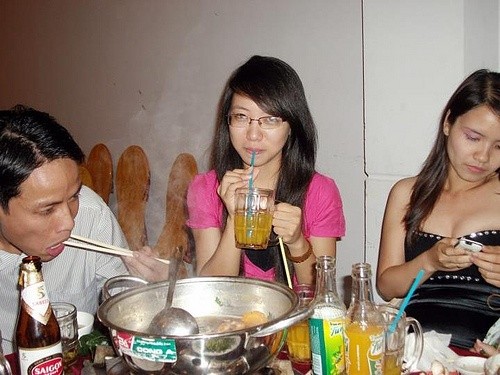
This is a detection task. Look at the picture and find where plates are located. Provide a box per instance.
[454,356,487,375]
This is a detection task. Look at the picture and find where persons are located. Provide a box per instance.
[469,319,500,375]
[186,55,346,297]
[0,104,170,358]
[376,70,500,351]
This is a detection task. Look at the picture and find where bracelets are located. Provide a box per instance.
[287,238,312,262]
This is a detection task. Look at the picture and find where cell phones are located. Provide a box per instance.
[455,237,484,254]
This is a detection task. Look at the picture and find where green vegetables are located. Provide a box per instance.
[195,336,234,353]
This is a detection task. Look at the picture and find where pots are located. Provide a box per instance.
[96,275,315,375]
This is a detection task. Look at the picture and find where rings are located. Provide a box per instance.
[457,266,460,268]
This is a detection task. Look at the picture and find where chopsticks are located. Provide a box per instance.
[62,234,171,265]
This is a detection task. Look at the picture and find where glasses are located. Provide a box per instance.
[227,113,289,128]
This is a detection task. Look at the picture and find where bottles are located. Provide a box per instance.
[13,255,66,375]
[0,330,12,375]
[307,256,346,375]
[342,263,386,375]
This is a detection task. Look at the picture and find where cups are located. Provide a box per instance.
[285,284,314,366]
[50,301,80,367]
[376,304,424,375]
[234,188,283,250]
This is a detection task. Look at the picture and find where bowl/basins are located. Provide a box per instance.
[58,311,94,340]
